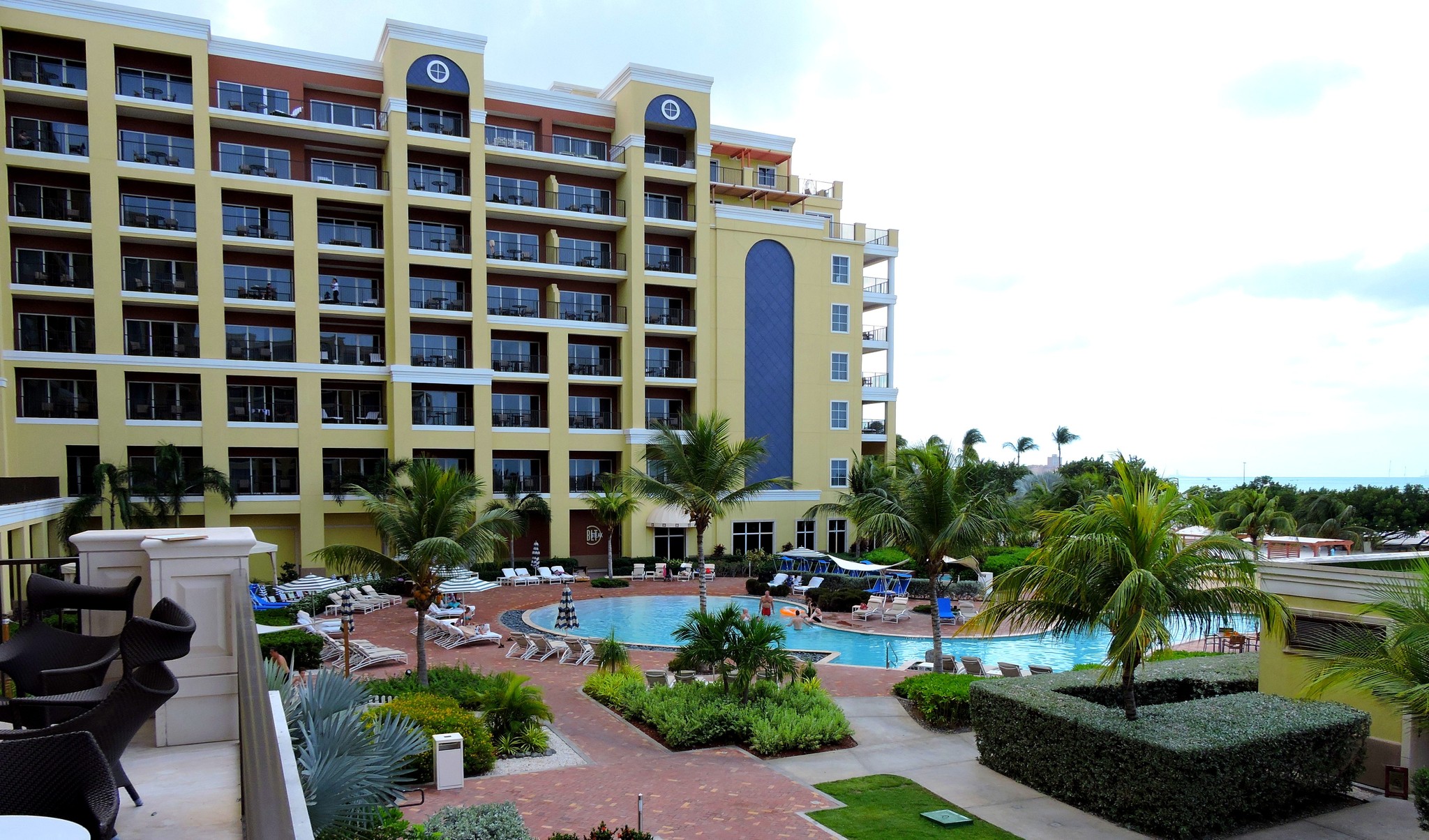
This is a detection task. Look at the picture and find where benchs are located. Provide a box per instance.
[293,555,1054,676]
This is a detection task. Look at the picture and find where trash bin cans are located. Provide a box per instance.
[978,572,993,594]
[431,732,465,790]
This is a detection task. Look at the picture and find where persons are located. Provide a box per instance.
[262,283,272,299]
[1331,547,1335,556]
[740,608,751,621]
[785,573,793,595]
[802,598,815,620]
[807,602,822,624]
[756,616,761,622]
[758,590,775,616]
[442,592,467,609]
[459,607,473,625]
[331,278,341,304]
[277,405,289,422]
[554,569,573,578]
[791,574,799,590]
[292,667,309,686]
[17,130,36,149]
[786,610,814,630]
[270,647,290,684]
[450,623,489,636]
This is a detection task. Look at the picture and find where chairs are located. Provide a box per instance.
[1243,632,1260,653]
[0,596,196,807]
[0,730,120,840]
[1219,627,1236,653]
[1203,631,1219,652]
[1,572,142,694]
[1223,635,1245,654]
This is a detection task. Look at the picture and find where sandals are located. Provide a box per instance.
[506,637,514,641]
[498,643,505,648]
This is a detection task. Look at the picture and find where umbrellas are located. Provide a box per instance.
[431,575,503,625]
[392,552,421,561]
[429,564,478,577]
[272,572,352,625]
[773,545,828,586]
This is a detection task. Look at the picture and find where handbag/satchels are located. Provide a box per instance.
[478,623,487,633]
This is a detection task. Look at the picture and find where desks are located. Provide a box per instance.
[0,814,92,840]
[1213,633,1246,653]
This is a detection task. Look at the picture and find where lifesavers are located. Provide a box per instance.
[779,605,806,617]
